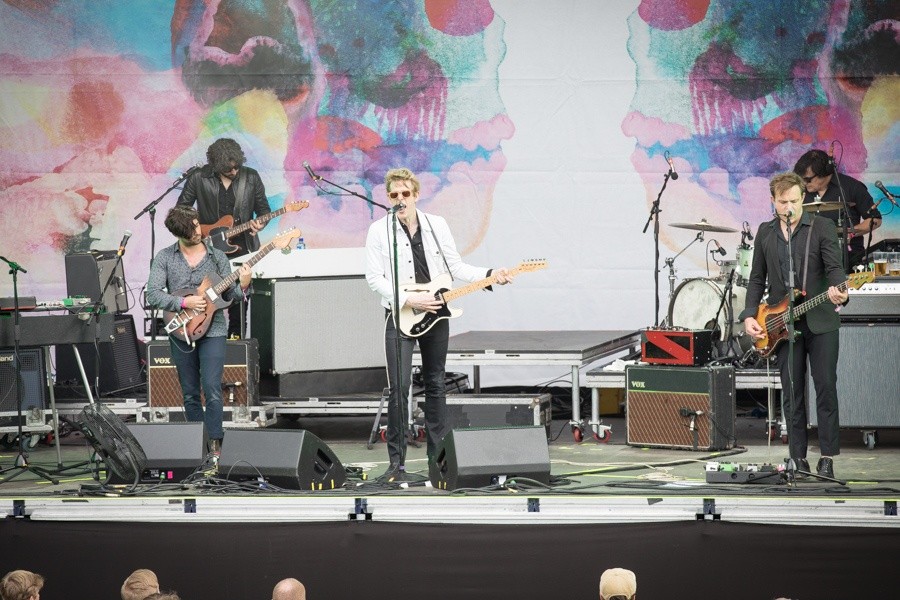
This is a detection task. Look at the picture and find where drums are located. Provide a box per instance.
[736,245,768,286]
[668,275,747,344]
[717,259,743,278]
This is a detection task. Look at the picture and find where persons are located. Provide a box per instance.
[0,569,45,600]
[364,168,513,474]
[121,568,181,600]
[272,578,307,600]
[795,149,882,274]
[737,174,850,482]
[148,207,253,474]
[599,568,636,600]
[176,139,270,339]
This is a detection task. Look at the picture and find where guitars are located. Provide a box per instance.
[163,225,301,348]
[750,263,876,358]
[200,199,309,256]
[390,258,549,339]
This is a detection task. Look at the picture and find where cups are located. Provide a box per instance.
[888,252,900,276]
[873,252,888,276]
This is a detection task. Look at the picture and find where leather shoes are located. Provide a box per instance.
[791,460,810,479]
[817,457,835,480]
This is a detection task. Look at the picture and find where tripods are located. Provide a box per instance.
[0,255,58,484]
[738,219,847,491]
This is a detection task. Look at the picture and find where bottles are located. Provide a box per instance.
[297,238,305,249]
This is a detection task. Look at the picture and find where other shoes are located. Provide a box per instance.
[209,440,221,455]
[375,464,405,481]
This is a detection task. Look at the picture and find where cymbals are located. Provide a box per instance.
[836,228,869,240]
[668,222,740,233]
[802,201,856,213]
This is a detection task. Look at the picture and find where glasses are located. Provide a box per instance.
[805,173,823,182]
[221,163,242,173]
[386,188,415,199]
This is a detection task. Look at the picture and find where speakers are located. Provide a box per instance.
[141,338,253,407]
[2,346,49,447]
[428,425,551,490]
[102,421,214,480]
[217,427,348,490]
[63,250,129,315]
[622,364,736,451]
[805,321,900,431]
[70,313,145,394]
[250,273,388,375]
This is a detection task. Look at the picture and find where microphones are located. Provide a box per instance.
[714,240,727,255]
[179,161,204,179]
[745,221,756,238]
[117,229,132,255]
[875,181,898,206]
[388,204,401,214]
[302,160,317,183]
[667,153,679,180]
[785,210,794,216]
[867,198,881,214]
[680,408,704,416]
[829,141,835,165]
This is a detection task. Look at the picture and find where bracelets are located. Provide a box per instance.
[182,298,187,309]
